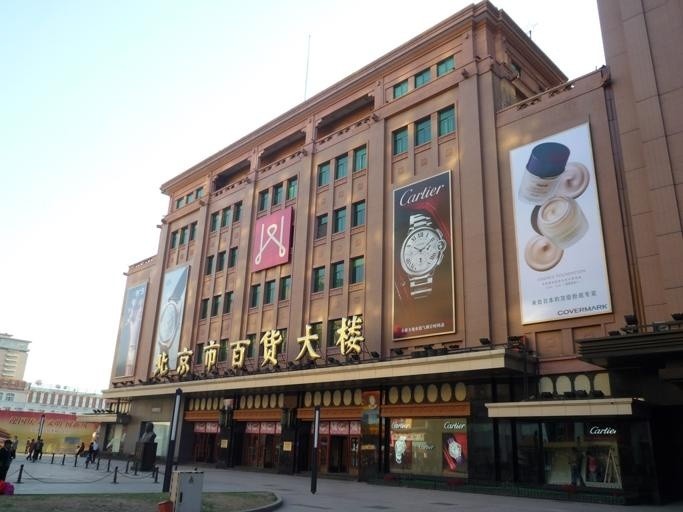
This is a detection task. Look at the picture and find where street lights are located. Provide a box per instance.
[308,403,321,495]
[37,413,45,441]
[162,386,183,492]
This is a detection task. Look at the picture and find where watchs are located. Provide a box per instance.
[156,269,187,354]
[393,434,409,462]
[445,437,464,464]
[398,212,447,301]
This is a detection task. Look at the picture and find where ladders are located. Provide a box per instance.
[602,447,622,490]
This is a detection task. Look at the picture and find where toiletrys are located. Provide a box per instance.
[517,142,570,204]
[532,195,588,249]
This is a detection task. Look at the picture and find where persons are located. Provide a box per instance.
[573,448,585,486]
[75,441,97,463]
[587,453,598,481]
[567,447,578,486]
[113,296,134,377]
[603,450,612,483]
[139,422,156,442]
[0,439,13,479]
[11,435,43,462]
[125,296,143,375]
